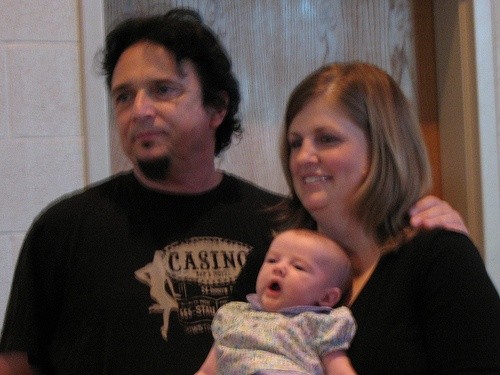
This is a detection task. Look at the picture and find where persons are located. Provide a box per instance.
[228,60,500,375]
[193,228,358,375]
[0,7,472,375]
